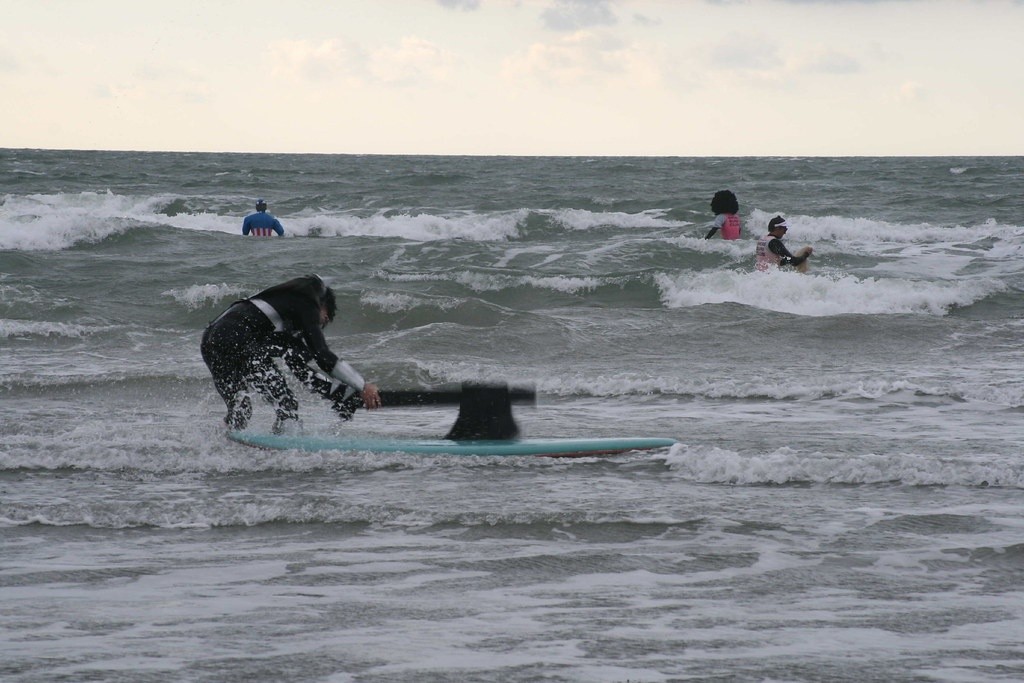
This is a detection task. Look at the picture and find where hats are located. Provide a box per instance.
[256,199,266,206]
[774,218,792,227]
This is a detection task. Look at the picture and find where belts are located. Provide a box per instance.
[250,296,285,332]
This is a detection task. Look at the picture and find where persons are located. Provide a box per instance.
[242,199,285,238]
[754,215,813,274]
[705,190,742,240]
[201,273,383,434]
